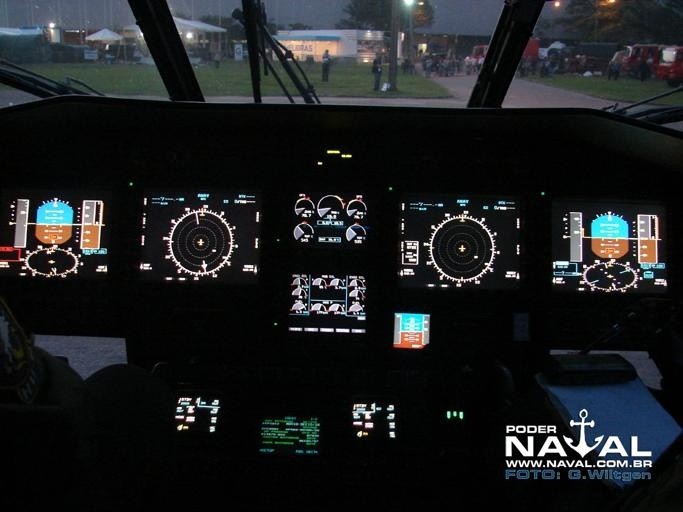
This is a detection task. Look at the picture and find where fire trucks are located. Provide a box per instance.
[464,37,541,66]
[619,43,683,88]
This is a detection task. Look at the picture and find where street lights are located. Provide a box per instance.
[404,0,417,75]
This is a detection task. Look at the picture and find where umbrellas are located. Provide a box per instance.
[84,28,123,57]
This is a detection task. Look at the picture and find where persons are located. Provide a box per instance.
[321,49,331,82]
[399,32,651,83]
[372,51,384,91]
